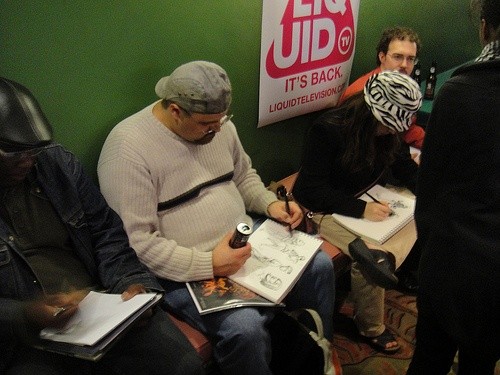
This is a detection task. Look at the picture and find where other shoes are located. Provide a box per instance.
[396,272,417,298]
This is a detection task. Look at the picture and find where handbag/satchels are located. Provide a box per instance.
[271,305,343,375]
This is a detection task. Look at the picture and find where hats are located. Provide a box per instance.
[154,61,232,114]
[364,71,423,132]
[0,77,53,147]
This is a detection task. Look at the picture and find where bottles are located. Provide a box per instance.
[424,64,437,100]
[412,60,421,89]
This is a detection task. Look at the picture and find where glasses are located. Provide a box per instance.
[384,53,420,65]
[184,108,233,134]
[0,146,45,160]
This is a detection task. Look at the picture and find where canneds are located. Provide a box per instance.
[229,222,253,249]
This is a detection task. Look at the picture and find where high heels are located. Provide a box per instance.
[361,328,399,352]
[349,238,397,290]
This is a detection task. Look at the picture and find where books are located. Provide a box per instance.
[186,219,324,315]
[331,184,416,244]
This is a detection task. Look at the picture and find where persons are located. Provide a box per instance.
[336,28,427,148]
[97,60,336,375]
[0,77,208,375]
[290,70,423,357]
[406,0,500,375]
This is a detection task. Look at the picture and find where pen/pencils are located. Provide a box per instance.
[285,195,292,236]
[365,192,381,204]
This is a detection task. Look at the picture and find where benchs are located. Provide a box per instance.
[170,168,348,359]
